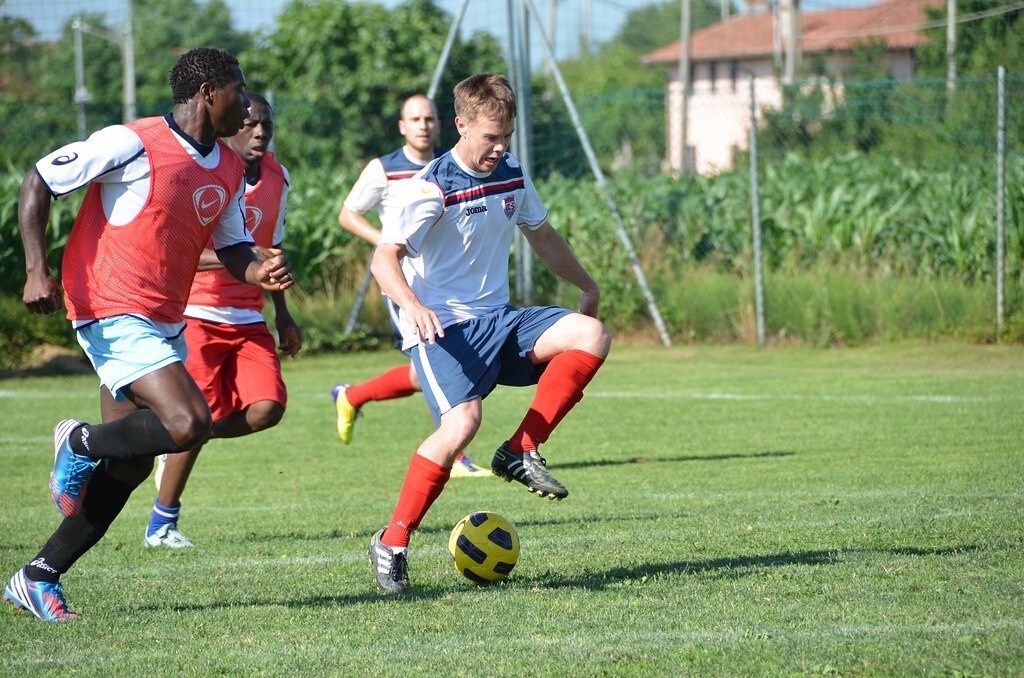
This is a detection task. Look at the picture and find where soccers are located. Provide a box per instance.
[448,510,521,585]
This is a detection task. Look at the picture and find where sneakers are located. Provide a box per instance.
[49,418,101,517]
[332,385,363,444]
[3,565,81,622]
[449,453,494,478]
[143,522,193,549]
[156,453,167,491]
[490,439,569,501]
[367,526,412,594]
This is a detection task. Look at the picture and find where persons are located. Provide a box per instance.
[98,89,292,549]
[4,47,294,620]
[328,96,499,477]
[370,73,611,594]
